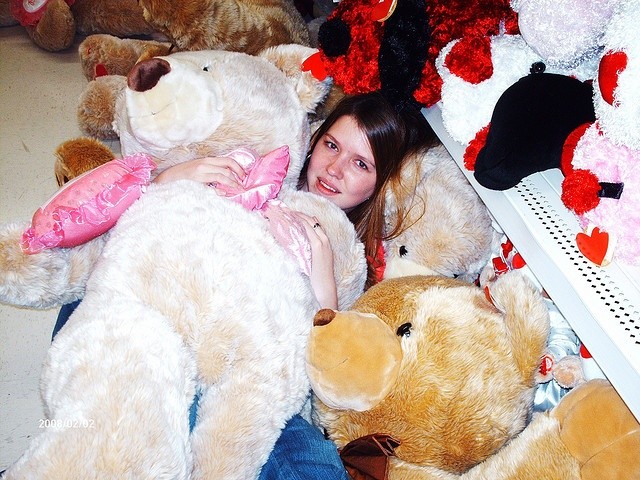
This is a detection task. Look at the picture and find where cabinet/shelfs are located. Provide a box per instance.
[418,99,640,424]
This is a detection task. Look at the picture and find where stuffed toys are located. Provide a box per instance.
[53,137,115,190]
[357,142,610,417]
[0,1,312,80]
[304,269,639,480]
[78,0,346,141]
[508,0,637,263]
[0,45,368,478]
[299,1,519,109]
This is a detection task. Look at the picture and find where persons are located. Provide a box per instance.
[50,90,442,479]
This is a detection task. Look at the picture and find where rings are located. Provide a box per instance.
[313,223,320,228]
